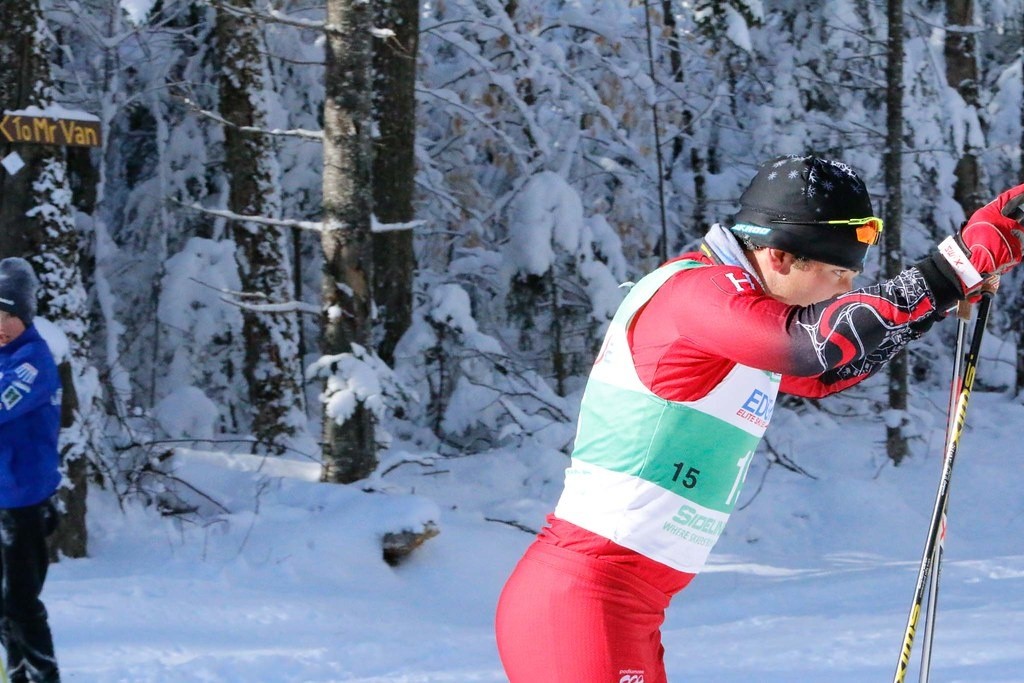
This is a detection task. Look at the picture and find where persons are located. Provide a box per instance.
[0,257,61,683]
[494,151,1024,682]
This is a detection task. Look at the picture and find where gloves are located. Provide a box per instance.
[932,183,1024,302]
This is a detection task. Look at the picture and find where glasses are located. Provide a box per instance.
[769,216,883,246]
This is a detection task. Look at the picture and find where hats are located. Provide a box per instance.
[728,154,874,272]
[0,257,38,325]
